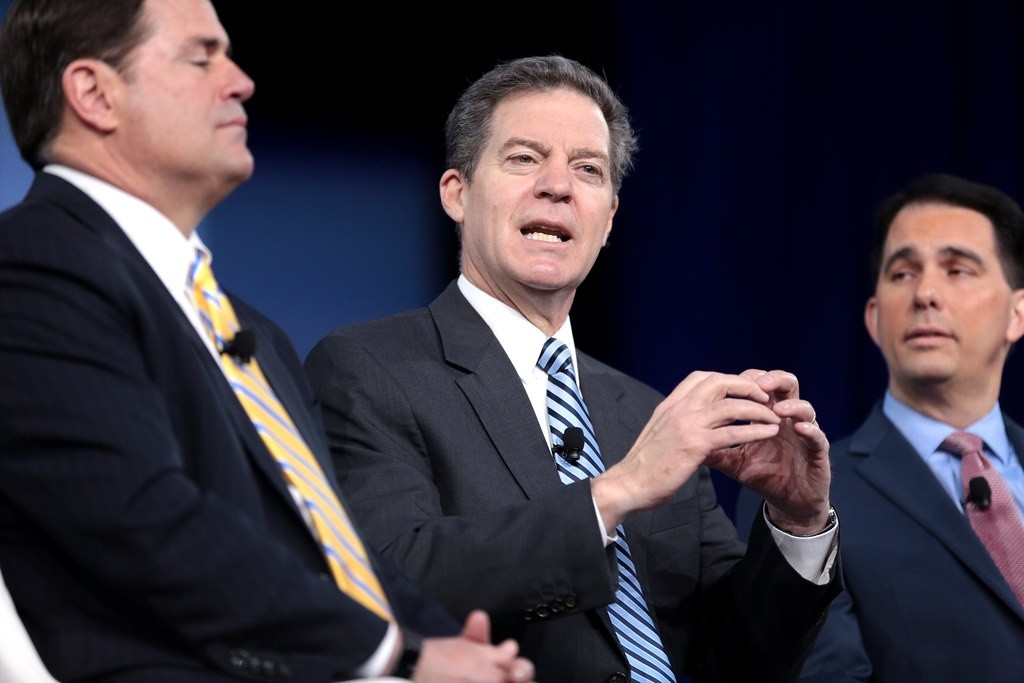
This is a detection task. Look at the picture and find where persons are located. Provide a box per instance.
[0,0,533,683]
[302,56,846,683]
[800,175,1024,683]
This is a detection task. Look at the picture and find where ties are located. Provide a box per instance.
[535,338,676,683]
[941,434,1024,603]
[189,247,396,627]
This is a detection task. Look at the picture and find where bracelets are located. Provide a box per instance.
[765,504,838,538]
[394,622,424,678]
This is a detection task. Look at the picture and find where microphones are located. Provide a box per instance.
[564,426,584,459]
[225,331,256,362]
[969,477,992,509]
